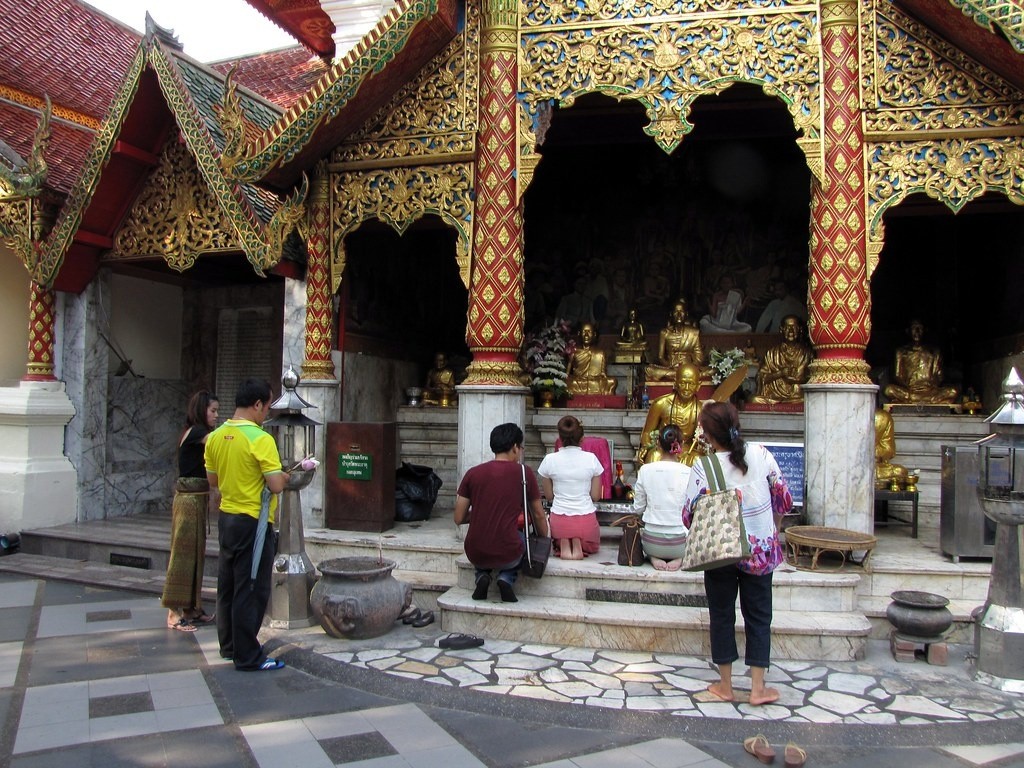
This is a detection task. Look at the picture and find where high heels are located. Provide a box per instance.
[744,734,776,764]
[784,740,807,768]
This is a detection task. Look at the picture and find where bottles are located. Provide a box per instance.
[612,462,632,503]
[642,385,649,408]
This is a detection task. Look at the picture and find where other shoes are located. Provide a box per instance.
[403,608,421,624]
[1,533,20,556]
[413,610,435,626]
[399,605,416,618]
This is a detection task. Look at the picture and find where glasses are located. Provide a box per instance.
[518,445,525,452]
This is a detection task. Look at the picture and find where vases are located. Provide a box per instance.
[534,388,568,408]
[715,384,743,408]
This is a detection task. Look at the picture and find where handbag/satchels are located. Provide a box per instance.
[681,454,752,572]
[522,535,551,579]
[610,514,645,566]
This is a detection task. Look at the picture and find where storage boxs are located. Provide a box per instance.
[324,422,397,533]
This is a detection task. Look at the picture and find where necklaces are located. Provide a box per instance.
[670,391,697,436]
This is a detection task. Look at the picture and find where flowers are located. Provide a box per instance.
[527,318,577,388]
[709,347,752,385]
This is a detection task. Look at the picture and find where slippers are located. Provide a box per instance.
[224,645,264,660]
[236,657,286,671]
[167,619,198,631]
[450,633,484,650]
[438,633,471,648]
[190,614,213,624]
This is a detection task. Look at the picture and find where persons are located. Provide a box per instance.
[453,422,548,602]
[421,353,458,406]
[616,309,648,346]
[637,363,703,467]
[161,390,219,632]
[538,416,605,559]
[204,378,290,672]
[875,406,908,489]
[748,314,813,404]
[643,303,716,381]
[682,401,794,705]
[632,425,692,571]
[882,319,959,404]
[566,322,618,395]
